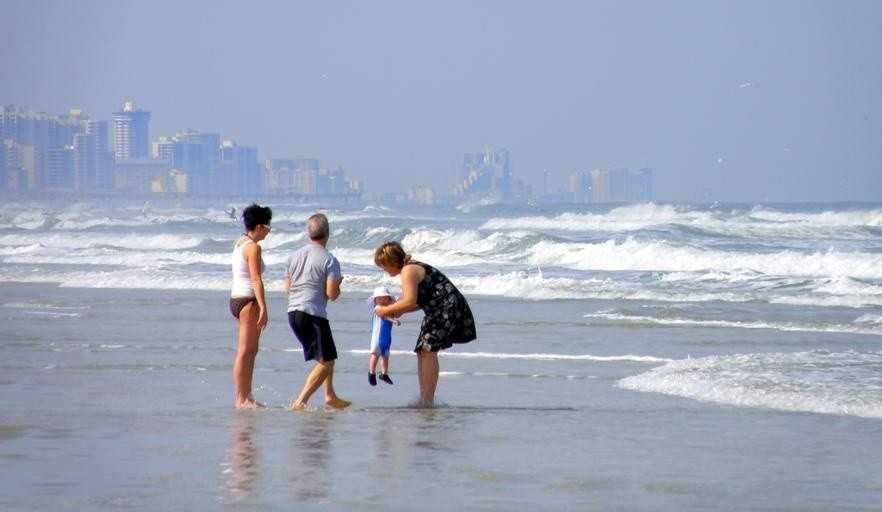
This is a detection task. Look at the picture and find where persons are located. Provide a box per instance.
[230,203,272,411]
[372,241,477,408]
[284,214,352,410]
[365,287,401,385]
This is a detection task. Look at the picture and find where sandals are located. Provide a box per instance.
[369,370,394,386]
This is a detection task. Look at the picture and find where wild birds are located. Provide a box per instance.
[222,207,239,220]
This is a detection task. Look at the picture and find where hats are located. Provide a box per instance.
[367,286,397,309]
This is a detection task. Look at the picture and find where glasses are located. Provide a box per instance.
[263,224,272,232]
